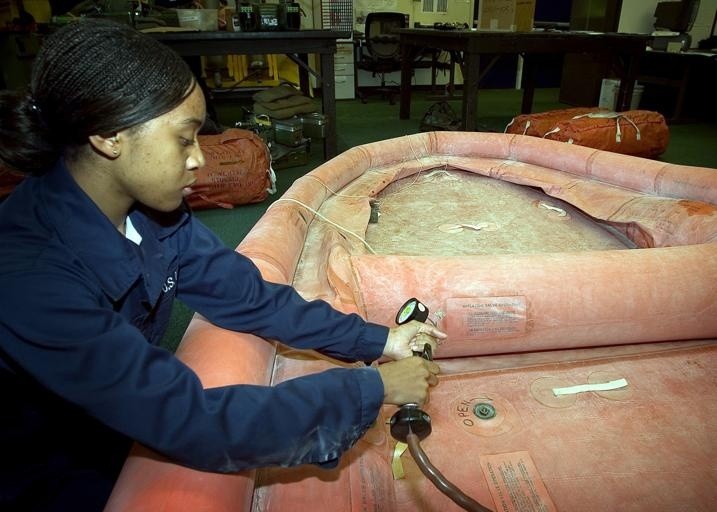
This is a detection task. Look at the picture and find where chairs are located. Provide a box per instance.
[355,12,415,105]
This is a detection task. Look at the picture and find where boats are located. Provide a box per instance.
[100,123,717,512]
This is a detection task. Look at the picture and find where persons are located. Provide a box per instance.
[0,21,447,512]
[150,0,204,11]
[216,0,230,31]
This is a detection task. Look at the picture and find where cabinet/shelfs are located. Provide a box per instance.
[333,42,356,100]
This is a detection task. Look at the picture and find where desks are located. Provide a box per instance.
[390,27,655,131]
[627,49,717,123]
[141,29,351,161]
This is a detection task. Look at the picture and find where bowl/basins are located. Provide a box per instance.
[173,8,220,32]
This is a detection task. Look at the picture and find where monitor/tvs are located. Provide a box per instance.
[653,0,701,32]
[473,0,572,29]
